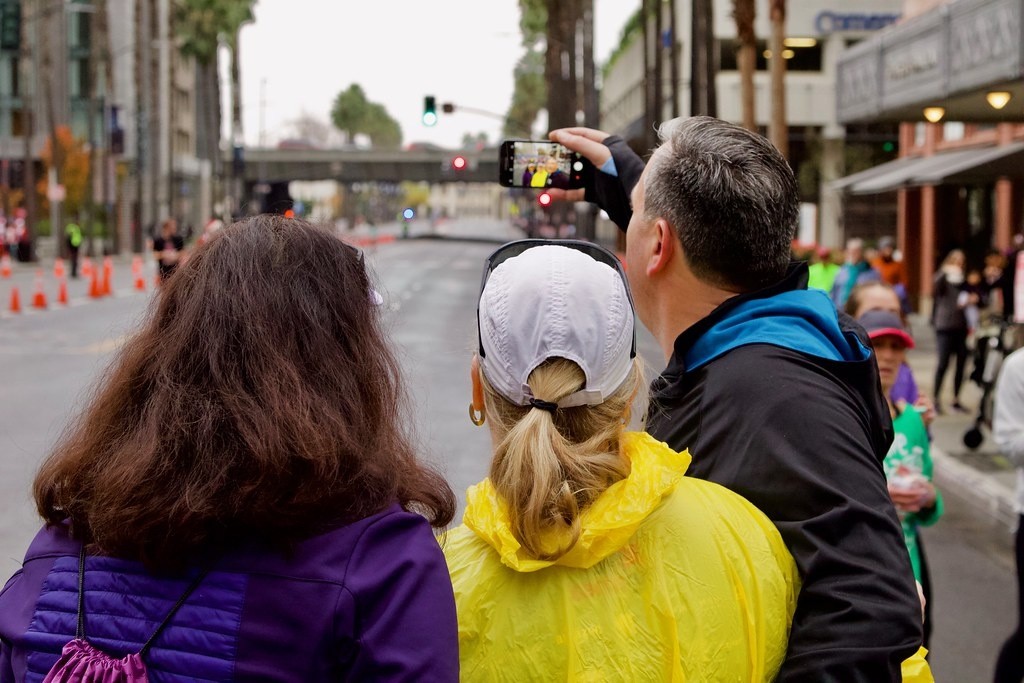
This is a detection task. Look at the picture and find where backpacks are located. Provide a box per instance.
[44,545,229,683]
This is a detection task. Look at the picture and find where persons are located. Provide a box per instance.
[1,214,464,683]
[427,238,938,683]
[64,215,83,278]
[548,114,929,683]
[515,157,571,189]
[791,230,1024,682]
[148,216,186,287]
[0,204,35,263]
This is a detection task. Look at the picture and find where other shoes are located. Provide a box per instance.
[952,401,970,414]
[934,402,944,416]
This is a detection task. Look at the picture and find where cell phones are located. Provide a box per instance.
[499,139,592,190]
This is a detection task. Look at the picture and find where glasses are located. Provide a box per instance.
[474,238,637,358]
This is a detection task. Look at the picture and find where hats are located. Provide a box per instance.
[860,311,916,350]
[479,244,634,408]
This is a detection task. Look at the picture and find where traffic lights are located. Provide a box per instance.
[424,95,437,126]
